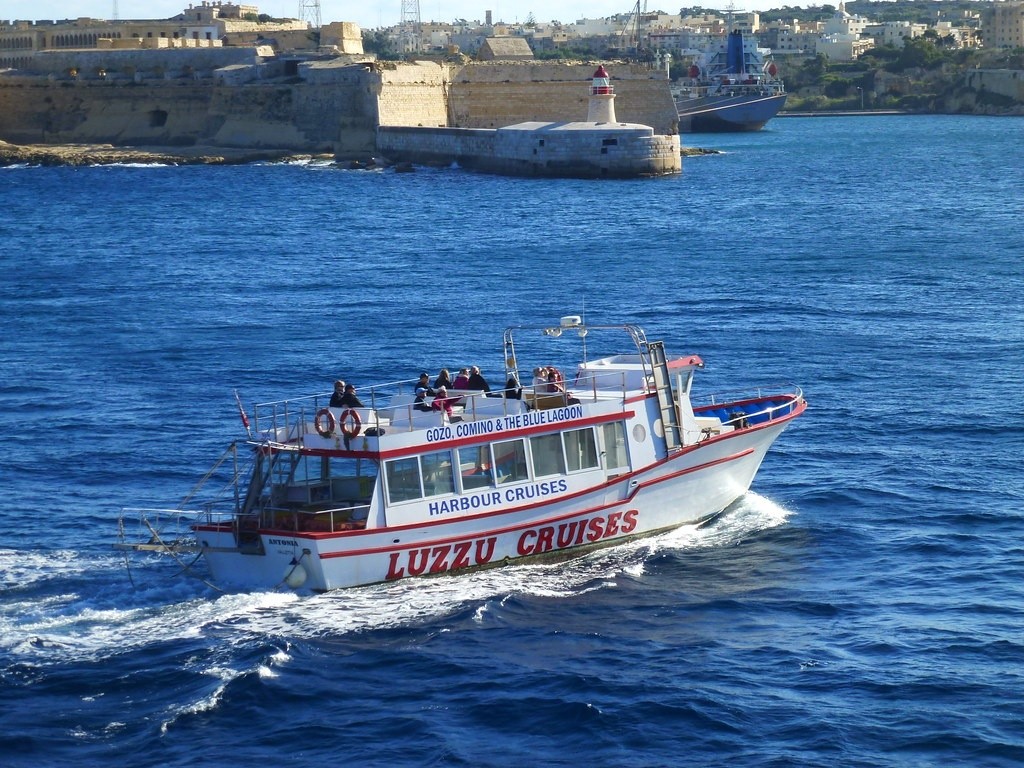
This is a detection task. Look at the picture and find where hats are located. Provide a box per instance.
[416,387,428,396]
[420,373,428,380]
[436,385,446,395]
[345,384,355,392]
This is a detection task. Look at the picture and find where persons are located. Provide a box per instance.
[503,376,525,404]
[328,378,366,411]
[529,363,581,404]
[411,363,493,419]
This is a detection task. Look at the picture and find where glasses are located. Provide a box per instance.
[340,386,345,387]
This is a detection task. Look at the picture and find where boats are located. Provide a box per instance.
[112,295,807,592]
[620,0,788,132]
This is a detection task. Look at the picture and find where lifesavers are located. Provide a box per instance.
[314,408,337,437]
[338,409,362,439]
